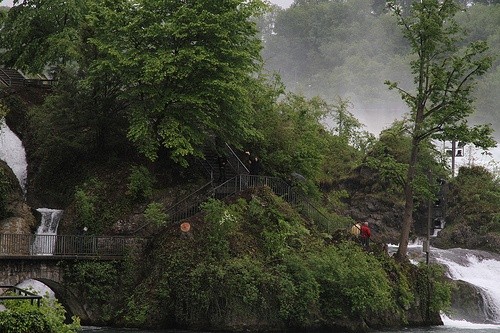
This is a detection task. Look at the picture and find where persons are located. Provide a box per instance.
[350,222,361,239]
[360,222,371,249]
